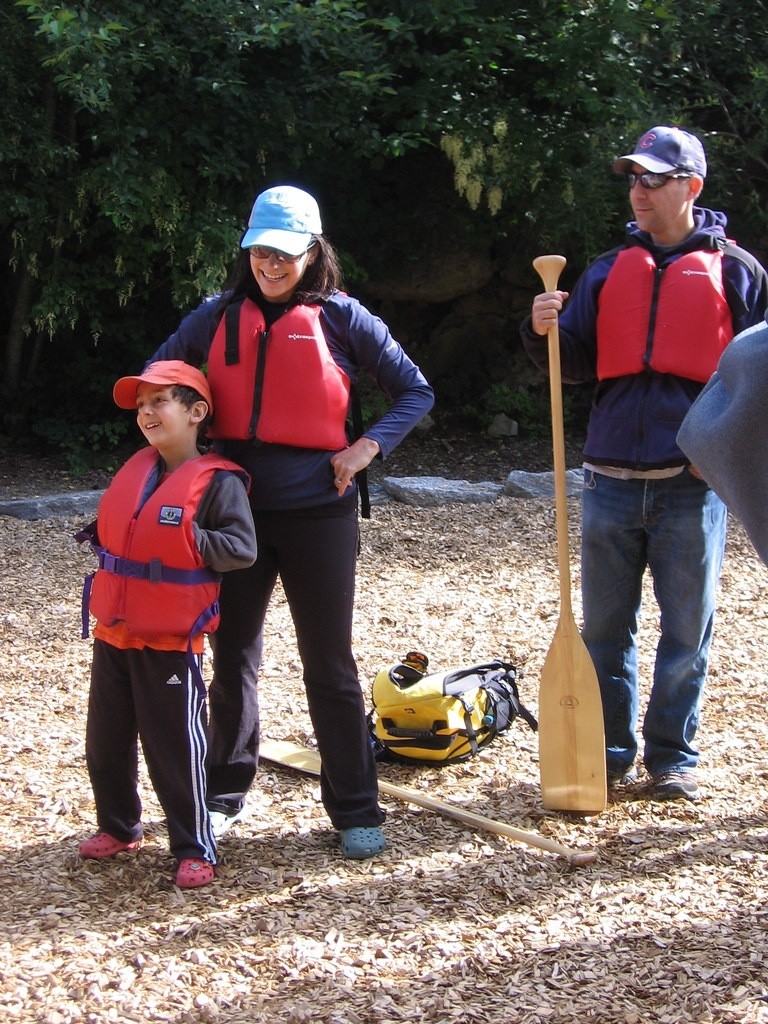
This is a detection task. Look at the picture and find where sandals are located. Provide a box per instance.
[78,832,143,858]
[340,825,384,856]
[176,858,215,889]
[207,808,251,838]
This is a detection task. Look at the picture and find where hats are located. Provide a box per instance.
[241,185,323,255]
[113,361,213,412]
[614,125,707,178]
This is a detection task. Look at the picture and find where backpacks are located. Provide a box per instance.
[370,654,539,767]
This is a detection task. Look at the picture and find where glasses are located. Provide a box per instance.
[625,172,698,189]
[248,242,316,264]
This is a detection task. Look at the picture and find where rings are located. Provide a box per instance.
[336,478,342,483]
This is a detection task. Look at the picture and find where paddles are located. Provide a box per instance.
[523,253,613,817]
[257,739,601,870]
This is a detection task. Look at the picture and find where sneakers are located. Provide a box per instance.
[654,773,699,801]
[608,759,636,789]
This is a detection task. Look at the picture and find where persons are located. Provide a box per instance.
[149,184,434,858]
[675,320,768,569]
[532,131,768,798]
[74,362,257,888]
